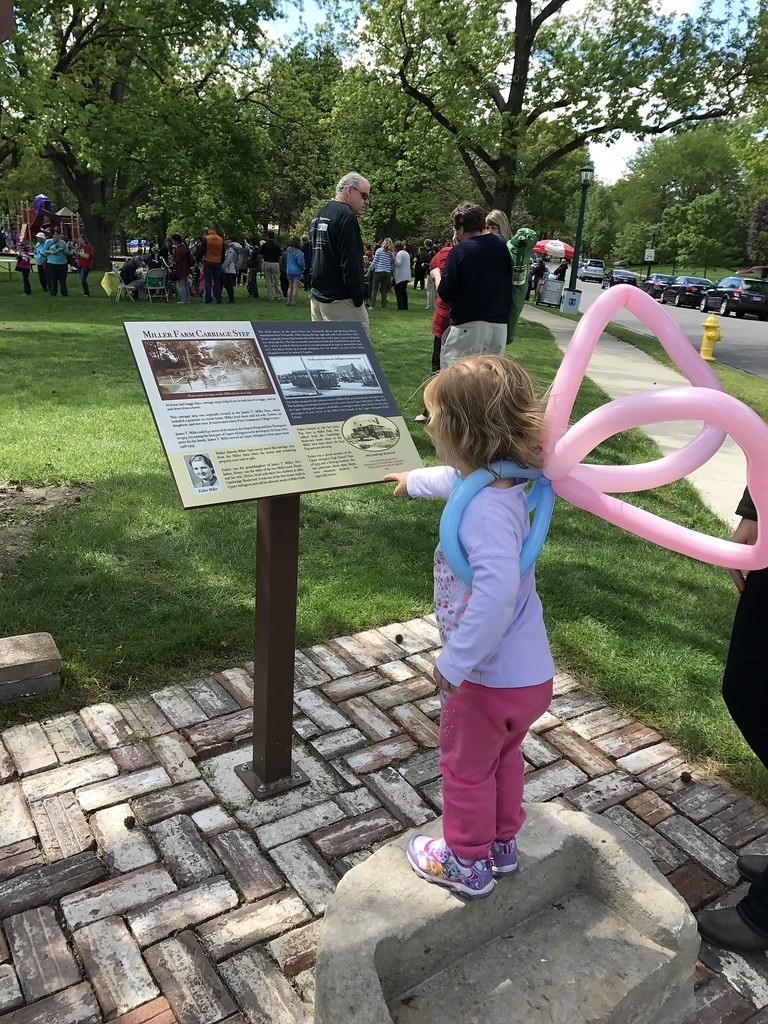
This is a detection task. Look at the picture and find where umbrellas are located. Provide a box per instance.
[533,239,574,273]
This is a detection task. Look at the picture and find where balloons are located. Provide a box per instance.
[438,283,768,582]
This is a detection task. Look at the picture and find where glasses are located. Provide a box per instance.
[346,186,368,200]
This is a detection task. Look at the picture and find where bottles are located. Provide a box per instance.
[544,271,549,280]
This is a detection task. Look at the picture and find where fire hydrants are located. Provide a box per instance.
[698,314,723,362]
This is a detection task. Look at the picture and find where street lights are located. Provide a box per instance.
[569,163,595,290]
[647,226,661,277]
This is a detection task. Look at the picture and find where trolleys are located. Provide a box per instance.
[533,278,565,310]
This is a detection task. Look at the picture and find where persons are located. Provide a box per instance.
[0,227,20,253]
[385,353,556,896]
[120,225,261,304]
[530,260,545,302]
[553,259,568,281]
[362,237,412,310]
[693,486,768,954]
[410,234,455,421]
[260,232,311,307]
[412,239,451,311]
[33,230,94,296]
[486,210,513,240]
[430,203,514,368]
[309,173,369,336]
[16,242,32,295]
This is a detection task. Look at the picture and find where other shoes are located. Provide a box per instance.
[369,306,374,311]
[694,905,768,954]
[177,299,190,304]
[736,854,768,883]
[22,292,29,296]
[83,295,88,297]
[411,414,430,423]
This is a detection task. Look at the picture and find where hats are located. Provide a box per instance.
[35,232,45,239]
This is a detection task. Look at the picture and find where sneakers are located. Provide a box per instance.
[487,835,519,878]
[406,833,495,899]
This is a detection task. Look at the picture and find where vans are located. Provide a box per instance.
[577,259,607,284]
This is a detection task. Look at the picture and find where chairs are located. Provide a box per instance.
[112,268,135,303]
[144,268,169,303]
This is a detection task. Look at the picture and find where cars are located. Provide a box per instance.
[638,273,676,299]
[660,275,715,309]
[699,275,768,321]
[600,269,637,290]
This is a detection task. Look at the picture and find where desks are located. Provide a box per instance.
[100,271,148,301]
[0,259,18,282]
